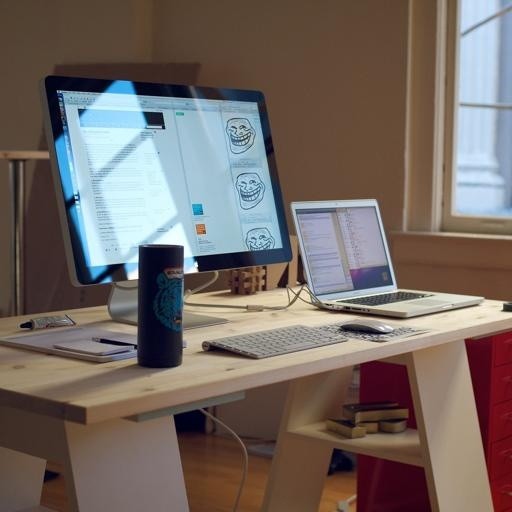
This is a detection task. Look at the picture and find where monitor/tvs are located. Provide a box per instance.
[37,75,293,331]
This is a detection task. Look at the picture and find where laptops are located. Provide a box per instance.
[289,199,485,319]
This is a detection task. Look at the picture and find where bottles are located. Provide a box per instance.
[136,243,186,367]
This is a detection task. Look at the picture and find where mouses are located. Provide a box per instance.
[340,318,394,334]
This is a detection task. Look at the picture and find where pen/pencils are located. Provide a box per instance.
[92,338,138,349]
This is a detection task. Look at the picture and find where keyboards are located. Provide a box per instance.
[202,324,348,359]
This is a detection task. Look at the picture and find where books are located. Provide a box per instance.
[53,338,133,356]
[2,326,186,362]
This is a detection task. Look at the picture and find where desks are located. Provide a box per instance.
[0,285,512,512]
